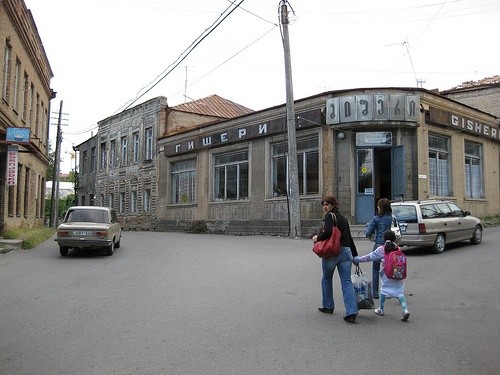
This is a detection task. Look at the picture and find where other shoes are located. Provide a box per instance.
[374,307,384,315]
[400,309,410,320]
[374,297,379,299]
[344,314,356,322]
[318,307,334,314]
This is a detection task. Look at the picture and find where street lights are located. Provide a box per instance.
[73,145,77,205]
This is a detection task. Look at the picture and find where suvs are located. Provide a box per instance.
[387,198,483,254]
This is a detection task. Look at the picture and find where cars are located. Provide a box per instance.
[54,205,122,255]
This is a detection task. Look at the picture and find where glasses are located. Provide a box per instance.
[323,203,330,206]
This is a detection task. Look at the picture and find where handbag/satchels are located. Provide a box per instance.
[350,264,375,309]
[391,215,402,245]
[312,212,341,258]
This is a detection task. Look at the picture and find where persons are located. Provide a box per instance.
[354,230,410,321]
[312,195,358,323]
[363,199,401,298]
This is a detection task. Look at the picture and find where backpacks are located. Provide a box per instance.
[383,245,407,279]
